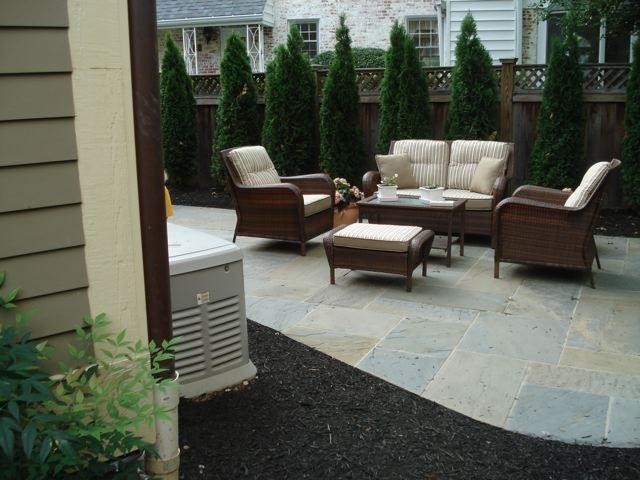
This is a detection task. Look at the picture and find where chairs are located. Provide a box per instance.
[495,159,623,290]
[220,144,337,257]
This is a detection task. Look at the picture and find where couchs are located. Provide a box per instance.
[363,139,516,246]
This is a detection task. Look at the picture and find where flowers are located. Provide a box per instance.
[332,177,363,204]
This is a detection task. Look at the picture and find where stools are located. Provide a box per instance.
[324,222,434,290]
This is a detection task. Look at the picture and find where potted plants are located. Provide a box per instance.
[375,171,399,202]
[419,184,446,203]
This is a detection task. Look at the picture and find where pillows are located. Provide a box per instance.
[374,152,418,190]
[471,156,505,194]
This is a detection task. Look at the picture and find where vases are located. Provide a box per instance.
[331,206,358,228]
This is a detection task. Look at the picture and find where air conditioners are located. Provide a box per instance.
[166,218,259,399]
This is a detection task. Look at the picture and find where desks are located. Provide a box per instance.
[357,192,470,269]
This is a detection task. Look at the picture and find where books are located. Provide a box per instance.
[418,197,454,207]
